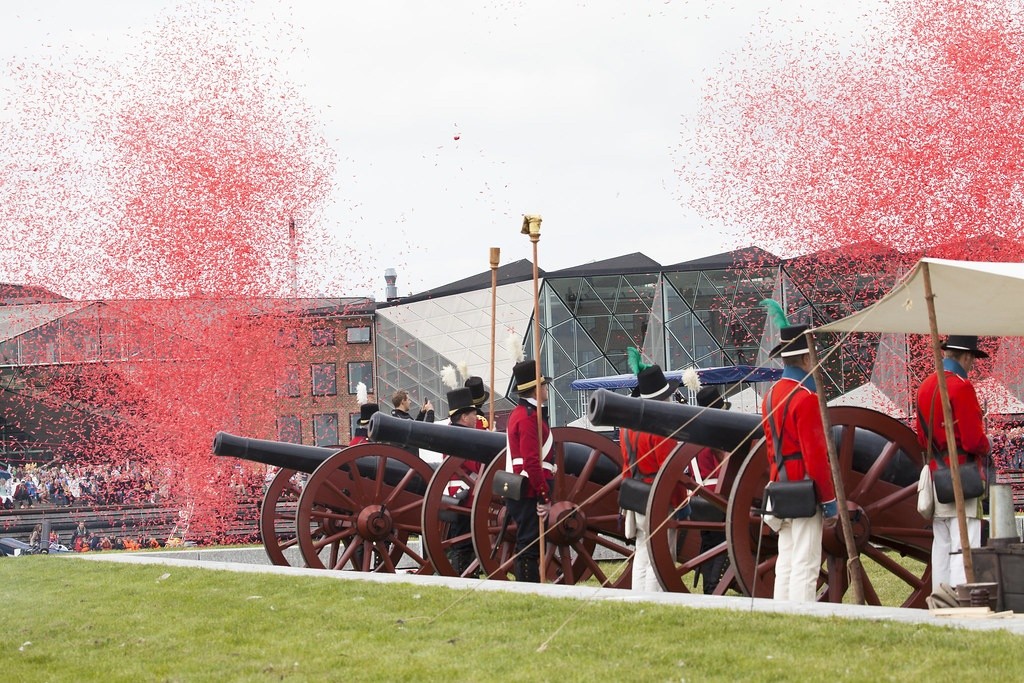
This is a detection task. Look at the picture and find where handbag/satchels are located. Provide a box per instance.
[768,479,816,519]
[918,465,935,520]
[492,471,527,503]
[617,479,652,515]
[933,463,986,505]
[440,498,462,521]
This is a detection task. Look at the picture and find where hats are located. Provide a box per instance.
[759,298,820,357]
[681,366,732,410]
[441,364,478,416]
[506,334,552,394]
[355,381,379,426]
[456,362,490,406]
[627,346,680,401]
[942,335,989,358]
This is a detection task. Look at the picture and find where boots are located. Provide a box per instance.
[372,543,388,569]
[515,556,538,582]
[348,546,364,571]
[449,548,479,578]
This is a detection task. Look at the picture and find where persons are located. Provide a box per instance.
[390,391,434,459]
[761,326,837,600]
[688,388,732,595]
[620,365,691,591]
[507,361,556,581]
[1001,420,1024,470]
[30,524,41,545]
[439,387,497,578]
[350,404,389,570]
[464,376,489,427]
[67,521,158,551]
[0,463,308,508]
[915,335,992,607]
[50,530,58,542]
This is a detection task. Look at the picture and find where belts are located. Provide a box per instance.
[773,451,802,463]
[643,473,657,479]
[512,456,558,476]
[703,478,718,486]
[448,480,466,488]
[938,447,966,456]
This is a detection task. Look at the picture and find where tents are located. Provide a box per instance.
[427,259,1023,652]
